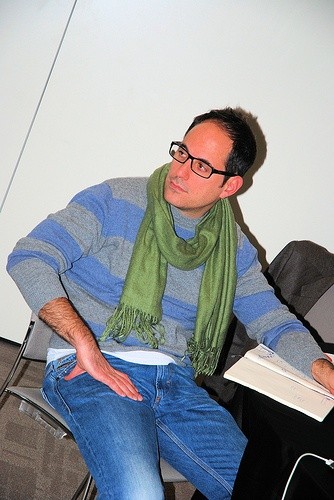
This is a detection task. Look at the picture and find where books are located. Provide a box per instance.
[223,343,334,422]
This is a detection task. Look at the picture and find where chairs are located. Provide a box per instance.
[0,313,191,500]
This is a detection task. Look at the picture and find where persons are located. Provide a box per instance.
[6,107,334,500]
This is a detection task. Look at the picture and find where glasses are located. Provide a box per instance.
[168,140,238,181]
